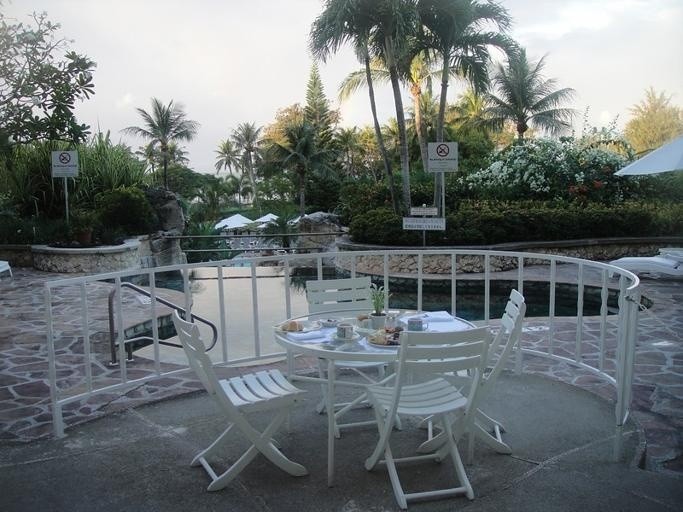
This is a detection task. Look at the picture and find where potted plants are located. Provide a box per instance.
[366,281,388,330]
[71,209,102,243]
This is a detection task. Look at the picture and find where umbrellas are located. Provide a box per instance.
[210,210,253,230]
[610,134,683,176]
[254,213,293,228]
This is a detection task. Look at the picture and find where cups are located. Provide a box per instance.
[407,317,428,332]
[336,322,353,340]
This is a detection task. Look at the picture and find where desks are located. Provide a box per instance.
[274,308,484,487]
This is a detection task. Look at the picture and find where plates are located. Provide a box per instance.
[280,320,321,333]
[330,331,360,342]
[365,337,402,348]
[319,315,344,327]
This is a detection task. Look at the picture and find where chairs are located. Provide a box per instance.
[414,281,527,461]
[167,310,310,493]
[301,276,399,438]
[603,249,682,285]
[363,323,489,508]
